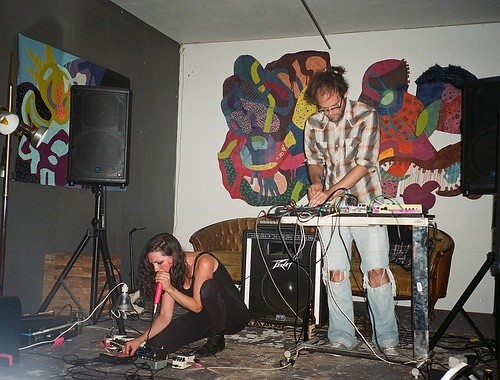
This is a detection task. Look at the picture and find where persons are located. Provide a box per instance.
[123,233,250,359]
[304,66,400,360]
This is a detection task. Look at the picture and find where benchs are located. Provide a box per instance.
[190,217,455,321]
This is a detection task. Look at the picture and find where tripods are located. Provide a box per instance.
[428,194,500,358]
[38,184,127,336]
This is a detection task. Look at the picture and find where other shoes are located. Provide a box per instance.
[197,335,225,357]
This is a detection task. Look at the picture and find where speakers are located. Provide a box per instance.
[67,85,132,189]
[240,230,329,328]
[462,75,500,194]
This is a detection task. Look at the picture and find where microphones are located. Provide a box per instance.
[153,282,162,314]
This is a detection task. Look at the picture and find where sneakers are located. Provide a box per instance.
[328,342,346,356]
[379,345,399,358]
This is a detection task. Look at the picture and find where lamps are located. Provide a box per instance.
[0,86,48,295]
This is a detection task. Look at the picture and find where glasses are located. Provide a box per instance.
[317,93,344,113]
